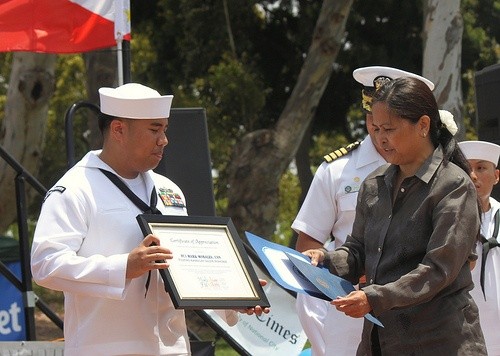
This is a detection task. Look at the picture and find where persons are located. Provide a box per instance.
[454,140,499,355]
[29,81,269,355]
[291,65,439,270]
[300,76,489,355]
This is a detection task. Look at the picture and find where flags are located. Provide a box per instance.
[0,1,132,53]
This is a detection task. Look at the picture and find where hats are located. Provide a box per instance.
[457,137,500,165]
[96,82,175,122]
[352,65,435,113]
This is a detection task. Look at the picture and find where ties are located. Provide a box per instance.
[474,211,500,301]
[98,169,170,299]
